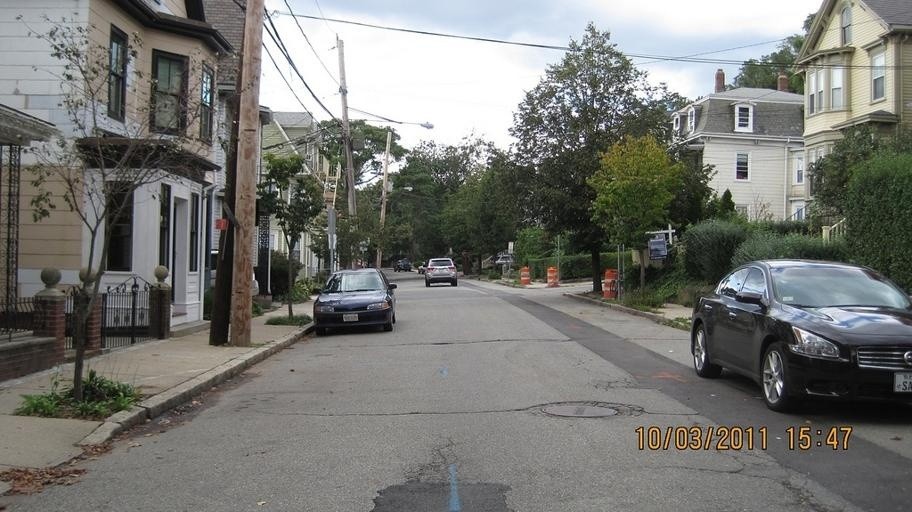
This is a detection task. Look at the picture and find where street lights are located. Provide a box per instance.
[330,32,437,268]
[374,185,412,269]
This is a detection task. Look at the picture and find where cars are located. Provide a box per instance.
[684,256,911,415]
[490,255,513,267]
[313,266,398,333]
[393,257,457,287]
